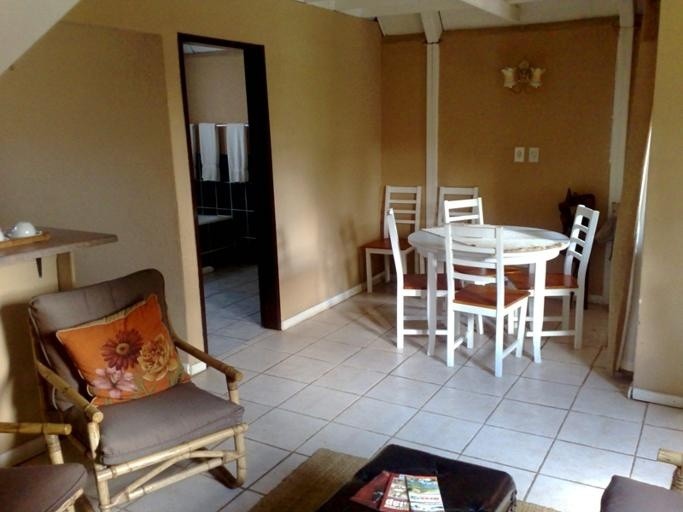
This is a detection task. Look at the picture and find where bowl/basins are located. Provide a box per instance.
[11,221,37,237]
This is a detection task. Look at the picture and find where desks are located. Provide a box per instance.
[0,224,115,472]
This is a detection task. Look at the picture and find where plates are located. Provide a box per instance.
[6,230,43,238]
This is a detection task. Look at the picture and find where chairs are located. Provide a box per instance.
[600,445,681,512]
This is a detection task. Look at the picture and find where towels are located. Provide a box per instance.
[188,122,247,182]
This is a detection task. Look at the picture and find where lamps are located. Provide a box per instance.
[501,58,546,93]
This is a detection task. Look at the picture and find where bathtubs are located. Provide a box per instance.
[197,213,228,224]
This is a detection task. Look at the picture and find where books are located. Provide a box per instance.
[404,474,445,512]
[379,471,410,512]
[350,470,390,511]
